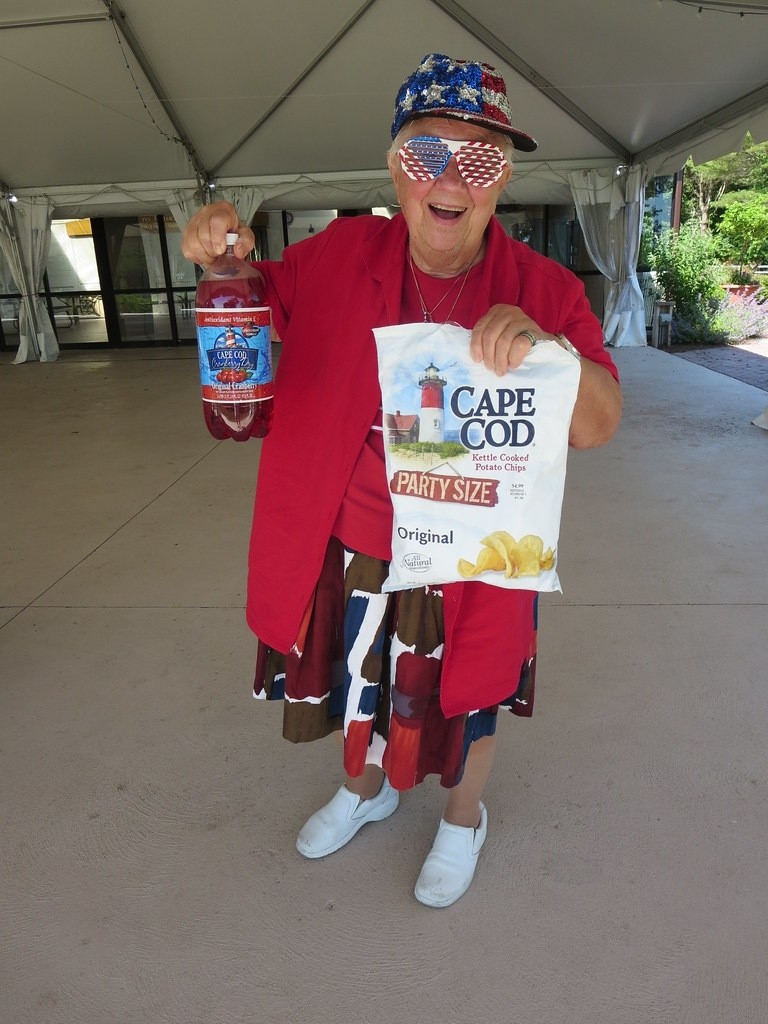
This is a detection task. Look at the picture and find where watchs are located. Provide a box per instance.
[554,331,582,361]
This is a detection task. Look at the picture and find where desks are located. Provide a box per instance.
[14,285,75,328]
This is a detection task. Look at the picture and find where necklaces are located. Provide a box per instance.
[408,238,482,324]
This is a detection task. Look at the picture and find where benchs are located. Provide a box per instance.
[647,300,675,348]
[7,305,85,329]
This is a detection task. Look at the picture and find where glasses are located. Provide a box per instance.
[396,136,510,189]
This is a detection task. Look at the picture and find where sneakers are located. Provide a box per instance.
[296,773,400,858]
[414,798,487,908]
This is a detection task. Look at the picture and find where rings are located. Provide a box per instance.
[518,331,536,347]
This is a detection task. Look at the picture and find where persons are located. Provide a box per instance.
[181,52,621,907]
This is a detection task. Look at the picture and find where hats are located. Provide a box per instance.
[390,53,538,152]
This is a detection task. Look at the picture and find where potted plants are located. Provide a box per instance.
[714,201,768,298]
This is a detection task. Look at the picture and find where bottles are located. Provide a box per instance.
[194,235,271,442]
